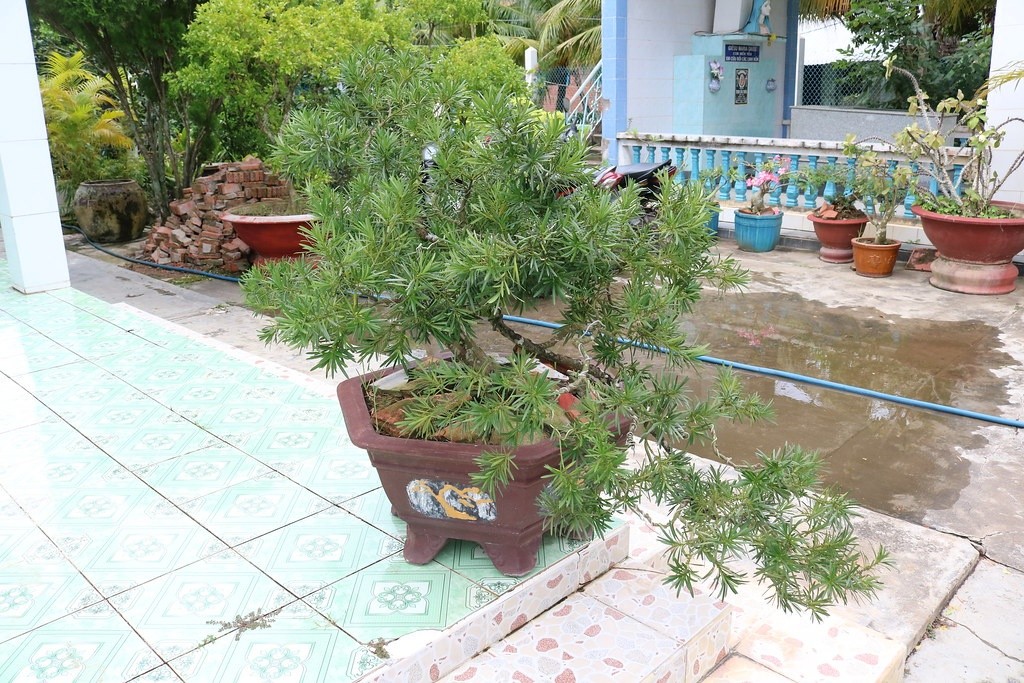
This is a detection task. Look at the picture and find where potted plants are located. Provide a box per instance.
[843,54,1024,297]
[247,30,894,615]
[843,151,908,279]
[693,168,738,234]
[160,1,388,258]
[797,161,872,264]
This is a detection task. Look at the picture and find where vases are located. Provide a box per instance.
[709,78,720,92]
[734,209,784,252]
[766,79,777,92]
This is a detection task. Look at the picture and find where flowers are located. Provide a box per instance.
[710,60,725,81]
[729,155,798,215]
